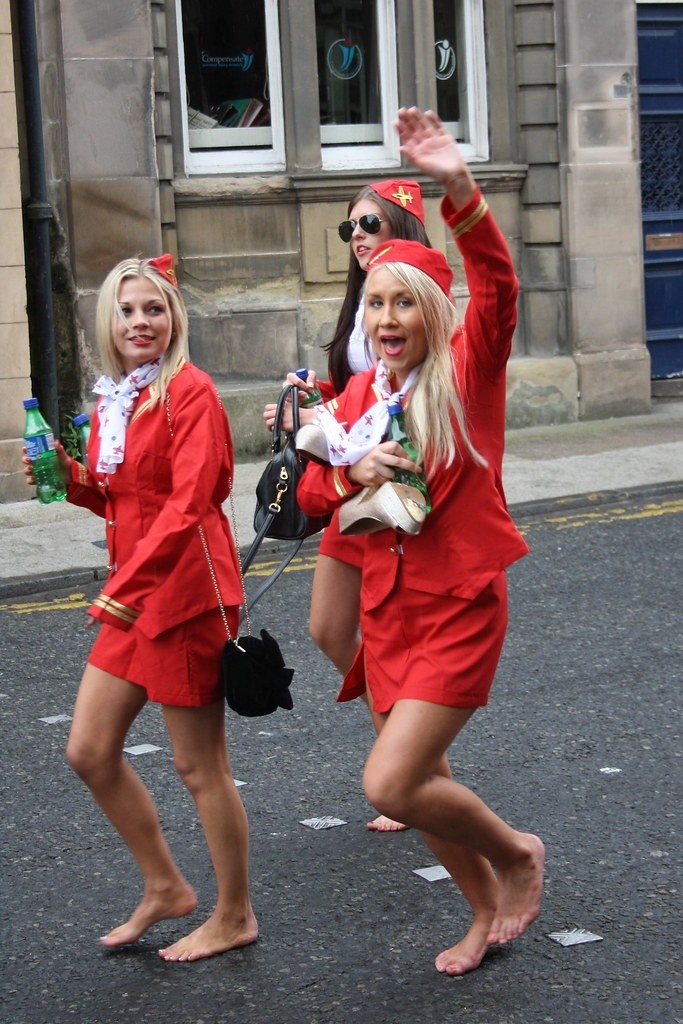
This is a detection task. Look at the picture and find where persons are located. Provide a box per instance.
[263,181,452,831]
[20,253,259,959]
[296,105,546,975]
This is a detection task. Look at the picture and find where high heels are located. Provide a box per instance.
[339,484,427,535]
[294,422,348,462]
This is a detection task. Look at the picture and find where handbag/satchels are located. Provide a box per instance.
[253,385,330,540]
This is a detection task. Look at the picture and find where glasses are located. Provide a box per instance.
[338,214,388,242]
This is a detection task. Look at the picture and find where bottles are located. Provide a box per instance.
[73,414,91,466]
[24,397,68,505]
[387,405,431,514]
[296,368,324,410]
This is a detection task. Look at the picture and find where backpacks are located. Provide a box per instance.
[221,630,295,717]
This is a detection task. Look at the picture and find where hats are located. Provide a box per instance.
[366,238,452,296]
[371,179,425,228]
